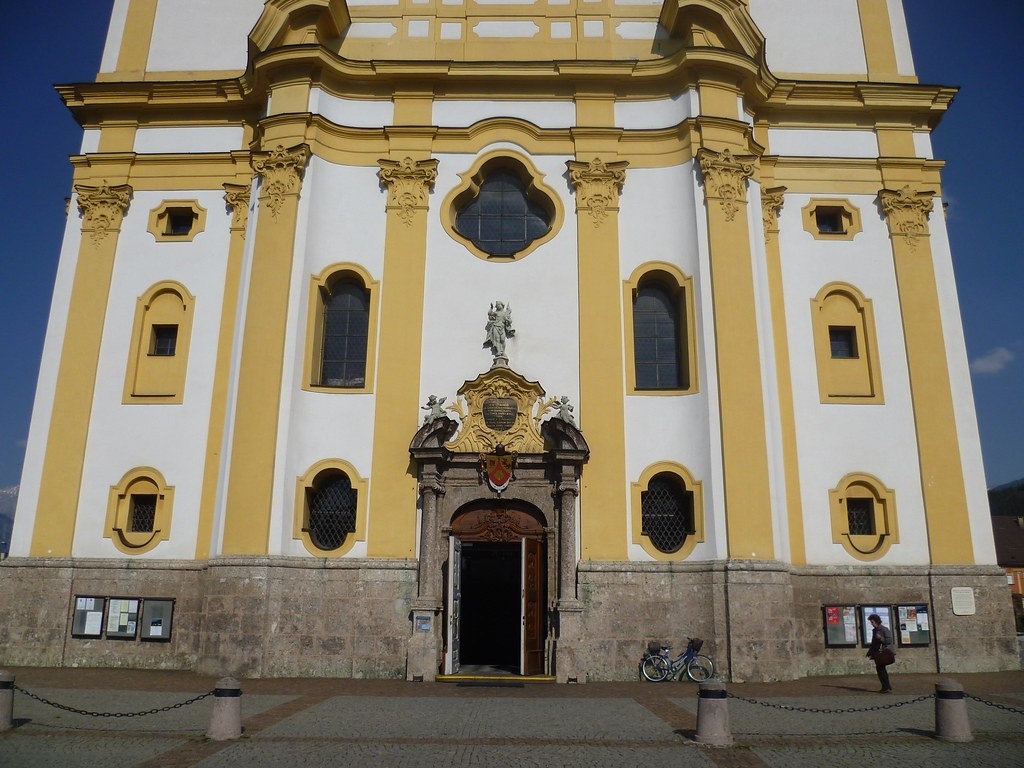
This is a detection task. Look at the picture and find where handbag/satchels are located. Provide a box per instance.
[878,638,895,665]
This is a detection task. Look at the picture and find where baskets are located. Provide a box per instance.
[691,640,703,651]
[648,643,660,652]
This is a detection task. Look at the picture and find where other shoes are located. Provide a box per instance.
[880,687,892,693]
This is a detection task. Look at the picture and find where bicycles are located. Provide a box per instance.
[639,637,713,682]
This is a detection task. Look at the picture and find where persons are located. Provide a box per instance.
[866,614,892,693]
